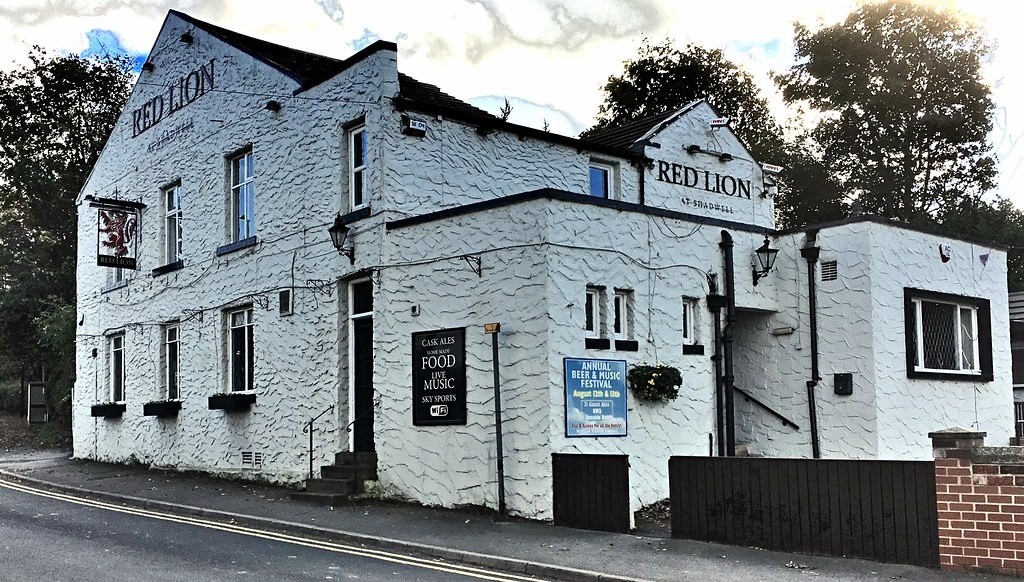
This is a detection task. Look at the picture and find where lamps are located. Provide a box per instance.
[751,233,780,287]
[328,212,355,264]
